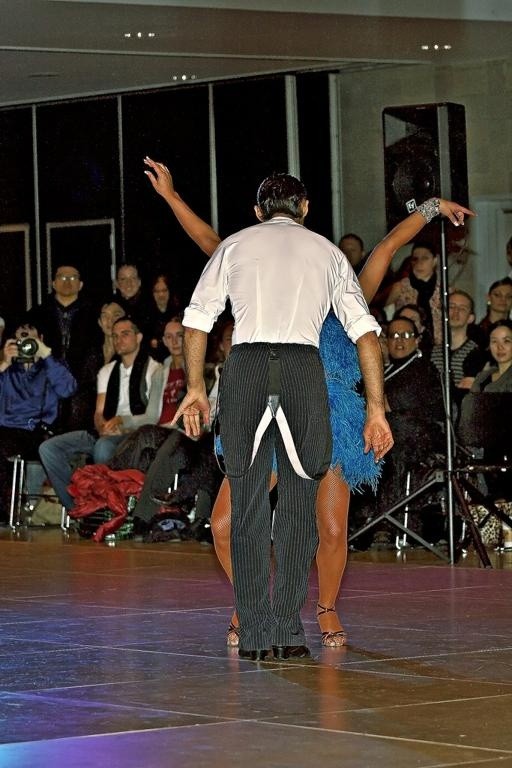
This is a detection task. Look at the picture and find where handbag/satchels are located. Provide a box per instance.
[143,513,193,543]
[32,487,76,526]
[464,505,511,546]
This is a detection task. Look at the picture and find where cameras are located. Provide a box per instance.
[10,338,38,363]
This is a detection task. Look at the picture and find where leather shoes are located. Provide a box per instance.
[134,516,148,539]
[196,518,213,545]
[238,645,310,660]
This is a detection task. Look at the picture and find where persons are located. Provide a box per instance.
[170,172,395,660]
[143,155,478,649]
[1,261,234,537]
[337,233,512,548]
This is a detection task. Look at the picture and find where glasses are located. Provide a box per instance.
[56,276,77,281]
[389,332,414,339]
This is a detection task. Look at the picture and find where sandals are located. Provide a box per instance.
[227,621,241,646]
[316,604,346,647]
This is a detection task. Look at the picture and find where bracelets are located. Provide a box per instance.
[416,198,441,224]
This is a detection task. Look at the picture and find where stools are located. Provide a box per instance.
[5,452,70,541]
[374,465,443,548]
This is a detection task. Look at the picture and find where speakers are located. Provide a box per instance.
[381,102,470,245]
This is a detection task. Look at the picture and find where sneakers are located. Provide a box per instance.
[350,527,375,552]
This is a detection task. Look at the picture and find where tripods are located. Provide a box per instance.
[348,221,512,569]
